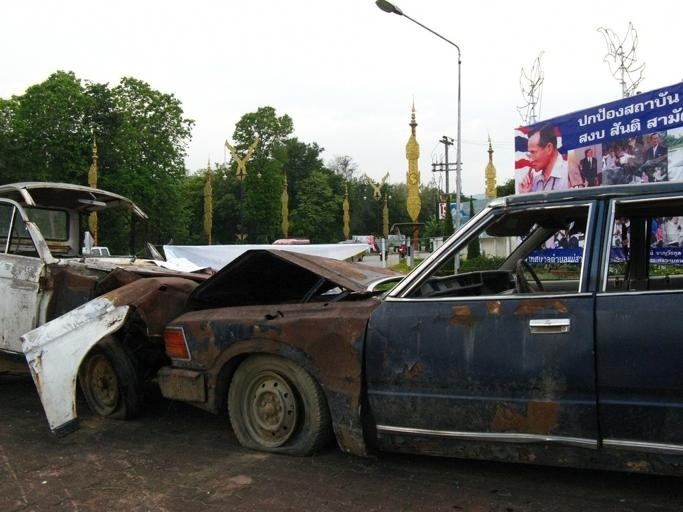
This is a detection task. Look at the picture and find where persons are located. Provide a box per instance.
[579,148,601,186]
[611,218,631,248]
[602,135,643,184]
[650,216,683,249]
[644,133,669,181]
[398,242,405,261]
[518,123,568,194]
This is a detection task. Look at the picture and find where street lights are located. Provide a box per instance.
[374,0,463,275]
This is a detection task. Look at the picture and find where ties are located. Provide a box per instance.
[588,158,591,167]
